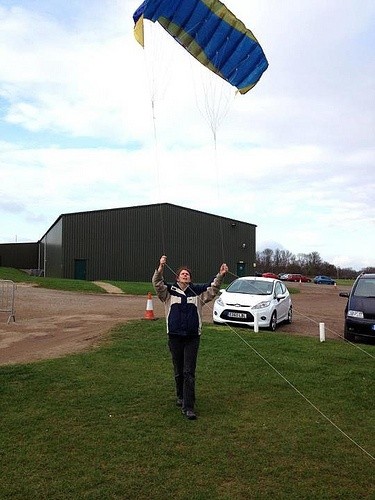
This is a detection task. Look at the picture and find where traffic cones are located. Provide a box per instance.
[139,292,159,322]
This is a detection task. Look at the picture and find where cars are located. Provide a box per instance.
[263,273,279,280]
[314,276,336,286]
[288,274,312,283]
[213,277,294,331]
[280,274,290,281]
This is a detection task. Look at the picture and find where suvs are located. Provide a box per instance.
[338,273,375,343]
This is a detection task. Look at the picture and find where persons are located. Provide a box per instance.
[151,254,228,420]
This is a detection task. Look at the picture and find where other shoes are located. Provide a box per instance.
[182,408,197,419]
[175,396,184,407]
